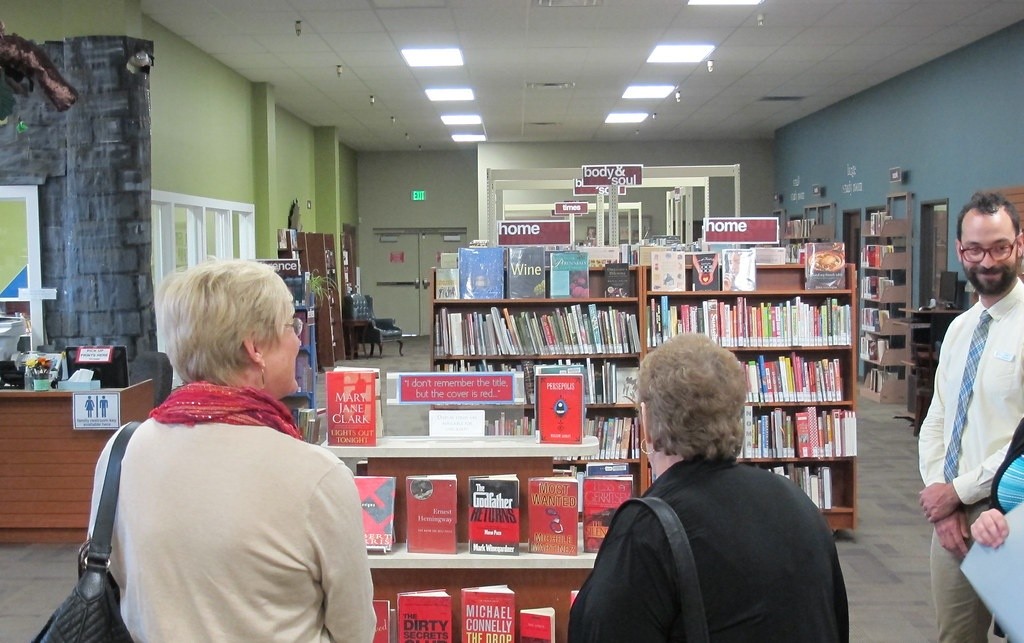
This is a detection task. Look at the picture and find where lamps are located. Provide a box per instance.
[291,0,764,150]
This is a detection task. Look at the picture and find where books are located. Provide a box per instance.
[290,211,897,643]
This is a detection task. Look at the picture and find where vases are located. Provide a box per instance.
[33,374,49,391]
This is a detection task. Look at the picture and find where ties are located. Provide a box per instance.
[943,309,992,485]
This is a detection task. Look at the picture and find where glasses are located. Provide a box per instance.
[959,233,1023,263]
[282,318,304,335]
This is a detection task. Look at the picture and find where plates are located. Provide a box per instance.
[809,252,845,271]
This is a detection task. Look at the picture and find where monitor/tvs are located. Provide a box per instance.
[67,345,130,389]
[0,361,26,390]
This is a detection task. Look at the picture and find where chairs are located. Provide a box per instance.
[344,293,405,359]
[913,313,961,435]
[127,348,173,411]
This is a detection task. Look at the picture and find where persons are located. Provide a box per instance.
[89,259,378,643]
[568,333,855,643]
[917,190,1024,643]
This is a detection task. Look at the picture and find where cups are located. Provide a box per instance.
[15,312,31,332]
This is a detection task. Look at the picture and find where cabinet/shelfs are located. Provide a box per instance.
[282,192,968,642]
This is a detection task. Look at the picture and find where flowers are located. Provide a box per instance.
[25,357,53,375]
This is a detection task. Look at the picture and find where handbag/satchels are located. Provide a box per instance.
[31,420,145,643]
